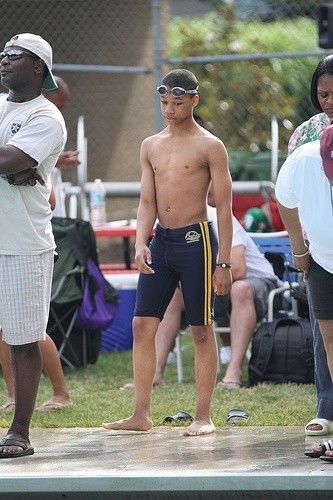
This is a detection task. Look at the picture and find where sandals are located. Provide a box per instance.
[305,418,333,435]
[304,440,333,456]
[320,450,333,458]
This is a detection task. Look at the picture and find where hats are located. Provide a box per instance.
[5,33,59,91]
[320,124,333,184]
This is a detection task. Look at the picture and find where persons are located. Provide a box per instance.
[0,172,74,414]
[42,75,83,166]
[0,32,68,459]
[274,54,333,462]
[118,182,282,392]
[101,67,234,437]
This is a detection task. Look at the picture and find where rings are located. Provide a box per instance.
[297,268,302,271]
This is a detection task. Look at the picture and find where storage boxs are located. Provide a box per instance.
[100,272,140,352]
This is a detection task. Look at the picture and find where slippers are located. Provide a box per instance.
[120,380,166,390]
[34,401,76,411]
[0,402,15,409]
[0,437,34,457]
[163,412,194,425]
[217,380,241,389]
[227,409,248,422]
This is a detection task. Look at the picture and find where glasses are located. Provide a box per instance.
[0,50,39,61]
[157,86,199,98]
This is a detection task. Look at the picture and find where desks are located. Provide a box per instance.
[93,226,155,270]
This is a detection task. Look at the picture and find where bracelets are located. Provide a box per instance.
[216,263,232,269]
[292,243,309,257]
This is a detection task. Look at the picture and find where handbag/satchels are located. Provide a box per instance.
[248,317,314,385]
[75,258,120,330]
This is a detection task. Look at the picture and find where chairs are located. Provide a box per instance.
[46,217,120,373]
[212,230,300,368]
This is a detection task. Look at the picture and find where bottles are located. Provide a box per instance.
[91,179,106,227]
